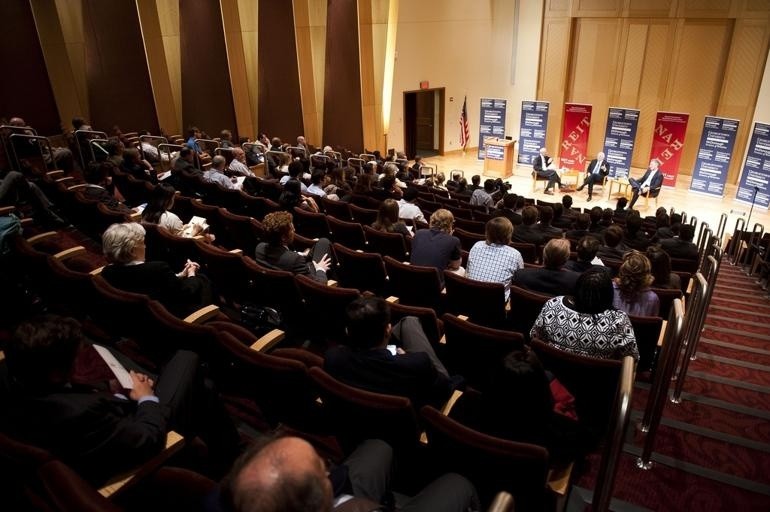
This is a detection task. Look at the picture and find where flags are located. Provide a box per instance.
[458,99,472,150]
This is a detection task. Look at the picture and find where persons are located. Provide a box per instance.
[650,227,680,249]
[464,217,525,304]
[613,197,635,220]
[572,233,608,274]
[240,138,252,148]
[394,189,429,225]
[324,147,333,154]
[378,165,404,194]
[453,175,461,185]
[470,340,580,438]
[458,178,467,192]
[602,207,614,223]
[204,156,237,192]
[503,194,519,208]
[301,158,315,173]
[84,166,147,216]
[278,164,303,184]
[408,177,419,184]
[254,210,340,286]
[267,138,284,152]
[363,165,377,175]
[470,179,504,209]
[673,213,683,225]
[533,148,566,195]
[220,131,232,143]
[188,128,214,159]
[320,300,437,406]
[528,266,642,361]
[331,167,350,189]
[296,136,306,149]
[0,348,241,475]
[626,159,663,208]
[434,173,446,189]
[357,175,376,193]
[227,147,258,179]
[590,207,607,233]
[407,209,465,279]
[565,214,592,240]
[368,161,379,173]
[105,135,126,161]
[467,175,483,191]
[244,174,265,196]
[92,222,213,314]
[596,228,626,262]
[539,206,564,238]
[410,156,425,172]
[619,216,649,248]
[343,167,357,181]
[645,244,683,292]
[552,203,571,228]
[612,249,661,319]
[511,206,546,244]
[1,169,72,232]
[291,151,303,164]
[283,144,289,149]
[138,132,180,159]
[627,208,643,219]
[104,164,126,203]
[373,151,383,160]
[275,154,290,173]
[365,198,410,237]
[228,432,488,511]
[517,238,573,299]
[136,184,215,243]
[120,146,161,184]
[424,177,436,188]
[563,196,580,217]
[308,170,327,198]
[379,154,399,173]
[71,117,108,148]
[278,177,320,212]
[256,134,270,146]
[576,151,610,201]
[516,196,528,209]
[646,207,670,224]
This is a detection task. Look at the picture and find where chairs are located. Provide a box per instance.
[671,258,698,271]
[15,153,97,208]
[457,216,486,232]
[160,227,204,267]
[293,233,332,265]
[100,203,130,222]
[383,254,441,300]
[321,198,350,219]
[584,170,606,198]
[112,166,167,202]
[442,268,505,323]
[456,228,486,249]
[399,218,415,234]
[510,243,538,263]
[371,297,441,347]
[333,243,383,282]
[321,213,364,246]
[444,311,525,372]
[349,203,379,223]
[347,175,414,209]
[168,193,191,215]
[671,271,690,292]
[534,339,624,439]
[311,365,413,430]
[197,241,245,293]
[443,203,472,221]
[507,285,549,323]
[435,194,459,208]
[297,272,362,336]
[217,335,307,410]
[241,256,293,303]
[425,396,550,503]
[621,313,664,363]
[474,209,494,220]
[155,302,285,361]
[416,196,441,212]
[630,183,658,211]
[136,218,167,252]
[94,272,152,320]
[250,218,315,253]
[190,199,218,228]
[0,218,86,285]
[650,287,682,316]
[292,207,324,236]
[532,168,553,196]
[219,207,259,247]
[196,178,281,214]
[0,434,198,512]
[363,226,405,255]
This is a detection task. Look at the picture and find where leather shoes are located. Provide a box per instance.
[587,197,592,201]
[577,186,584,191]
[543,189,553,195]
